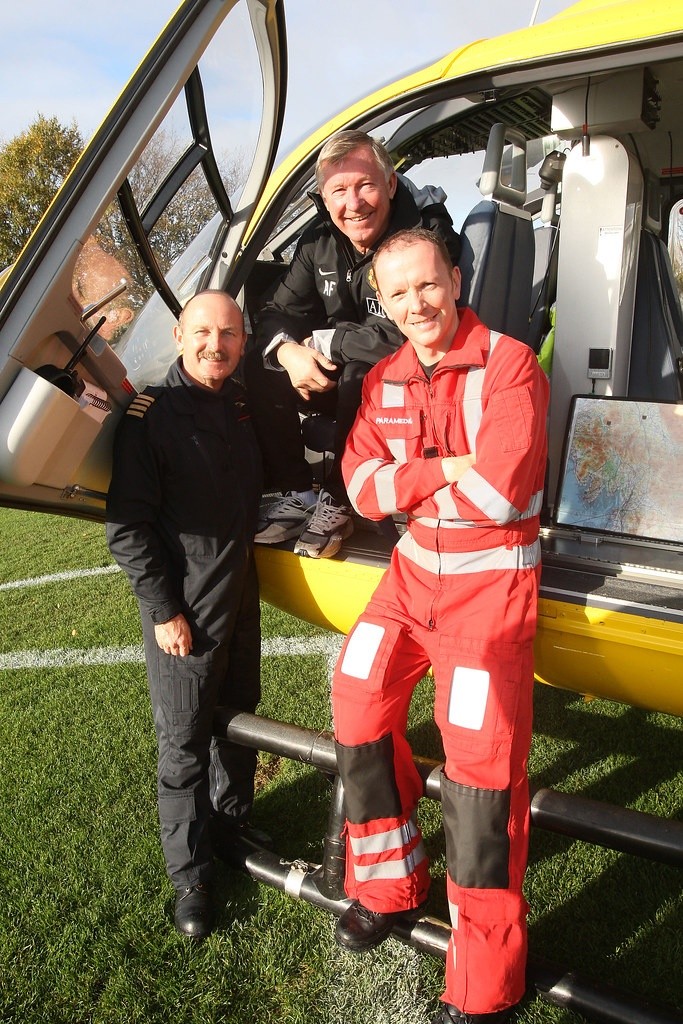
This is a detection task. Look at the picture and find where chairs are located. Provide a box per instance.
[301,123,536,455]
[528,214,559,355]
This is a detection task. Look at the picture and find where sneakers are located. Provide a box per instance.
[253,492,317,545]
[293,487,355,559]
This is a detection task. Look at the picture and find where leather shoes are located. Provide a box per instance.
[232,819,282,853]
[173,878,214,937]
[333,898,430,952]
[431,1002,510,1024]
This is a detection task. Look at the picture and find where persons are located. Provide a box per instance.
[241,127,467,560]
[106,288,276,938]
[330,227,553,1024]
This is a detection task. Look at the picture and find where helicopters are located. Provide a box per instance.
[0,0,683,1024]
